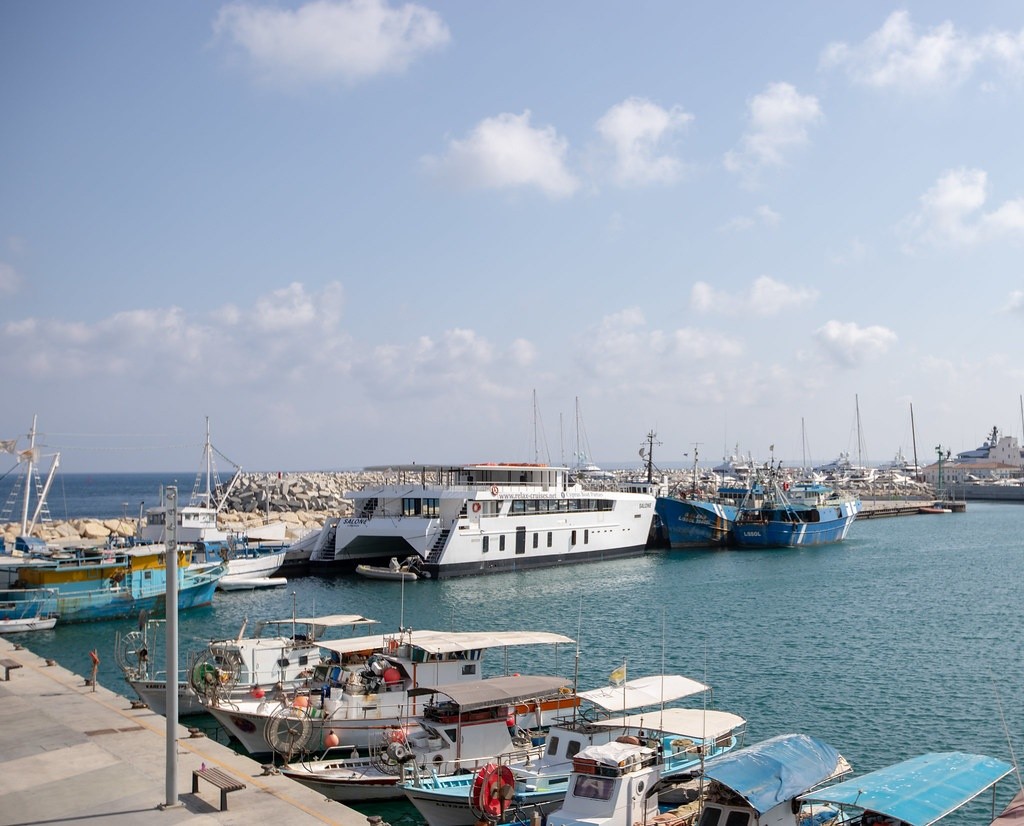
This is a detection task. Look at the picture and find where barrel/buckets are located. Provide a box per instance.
[321,651,340,697]
[670,739,710,760]
[330,688,343,701]
[310,695,322,709]
[530,734,546,747]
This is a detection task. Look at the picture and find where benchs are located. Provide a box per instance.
[191,767,246,811]
[0,658,23,681]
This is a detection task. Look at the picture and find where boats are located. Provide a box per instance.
[1,386,1024,826]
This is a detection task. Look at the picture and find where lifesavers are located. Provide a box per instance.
[472,503,481,513]
[784,481,789,491]
[492,486,499,495]
[617,735,641,745]
